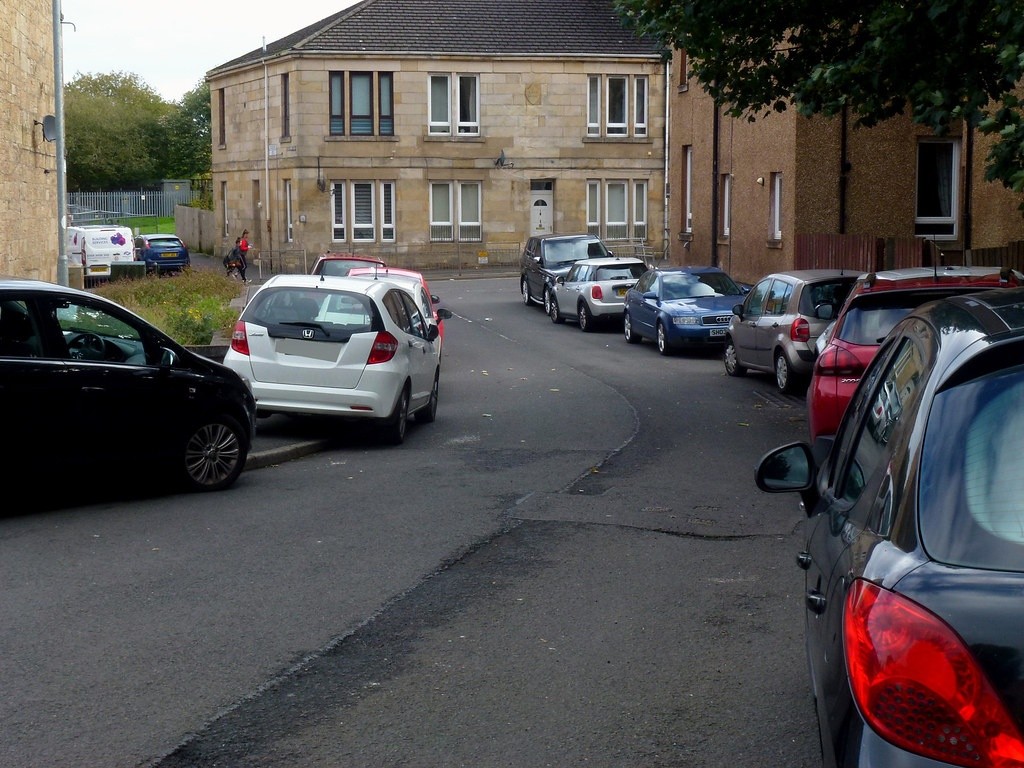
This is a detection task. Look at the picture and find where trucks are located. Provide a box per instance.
[66,225,137,286]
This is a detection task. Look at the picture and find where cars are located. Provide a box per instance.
[223,274,440,447]
[316,272,452,360]
[310,249,387,276]
[623,266,752,356]
[344,267,445,343]
[722,269,868,394]
[550,256,649,331]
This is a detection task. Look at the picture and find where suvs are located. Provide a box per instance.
[519,232,615,315]
[805,267,1024,446]
[753,285,1024,768]
[806,265,1024,364]
[0,274,256,503]
[134,233,190,278]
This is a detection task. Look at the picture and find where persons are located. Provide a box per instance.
[226,237,252,285]
[232,228,252,281]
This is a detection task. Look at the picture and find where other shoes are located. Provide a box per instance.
[242,279,252,282]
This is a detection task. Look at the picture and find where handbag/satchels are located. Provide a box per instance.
[223,256,230,268]
